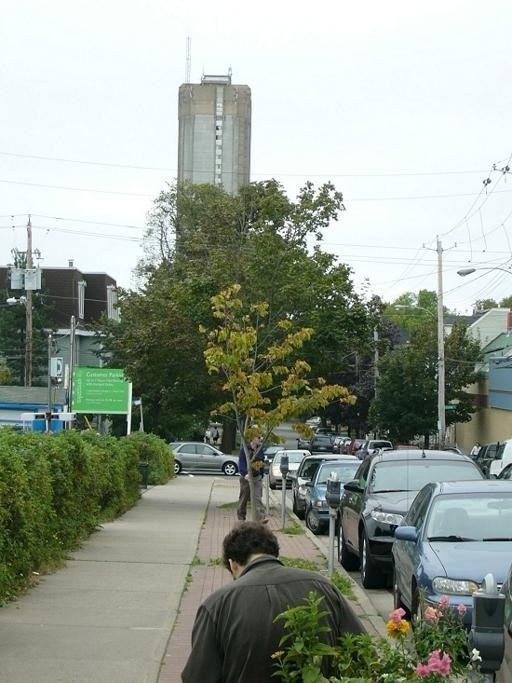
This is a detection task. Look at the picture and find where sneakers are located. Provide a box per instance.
[237,511,246,520]
[262,518,269,524]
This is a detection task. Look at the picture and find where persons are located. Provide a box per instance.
[181,522,378,683]
[345,439,356,455]
[205,425,219,445]
[237,435,270,524]
[470,441,482,456]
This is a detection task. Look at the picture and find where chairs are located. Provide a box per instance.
[436,507,472,537]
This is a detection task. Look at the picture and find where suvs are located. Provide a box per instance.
[169,440,240,475]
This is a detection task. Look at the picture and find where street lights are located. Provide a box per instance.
[5,295,33,386]
[394,303,446,450]
[456,265,512,277]
[133,395,143,432]
